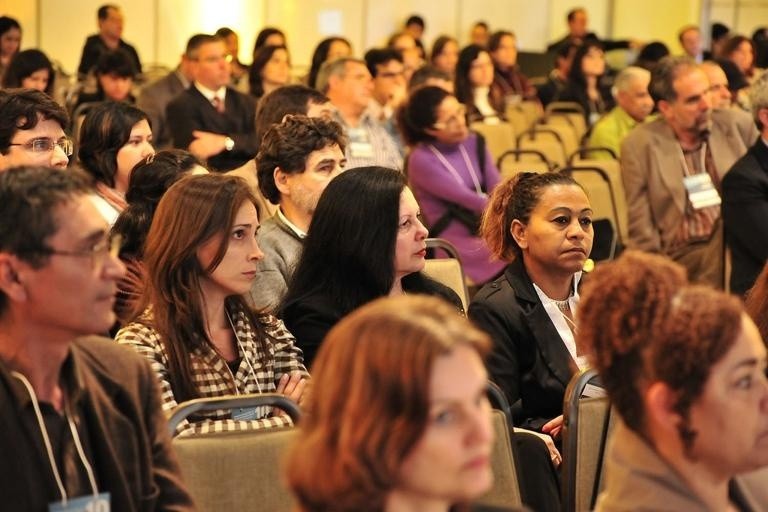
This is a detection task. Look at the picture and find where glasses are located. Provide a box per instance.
[5,137,74,157]
[10,233,121,270]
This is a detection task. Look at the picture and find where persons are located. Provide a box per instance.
[267,165,563,512]
[2,163,201,511]
[574,247,768,512]
[109,172,314,436]
[281,291,527,512]
[465,169,595,482]
[4,2,767,344]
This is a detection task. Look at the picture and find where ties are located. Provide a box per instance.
[211,96,225,112]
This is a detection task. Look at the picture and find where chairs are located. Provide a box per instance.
[165,395,307,512]
[460,379,529,512]
[417,235,471,318]
[469,94,630,258]
[561,368,615,512]
